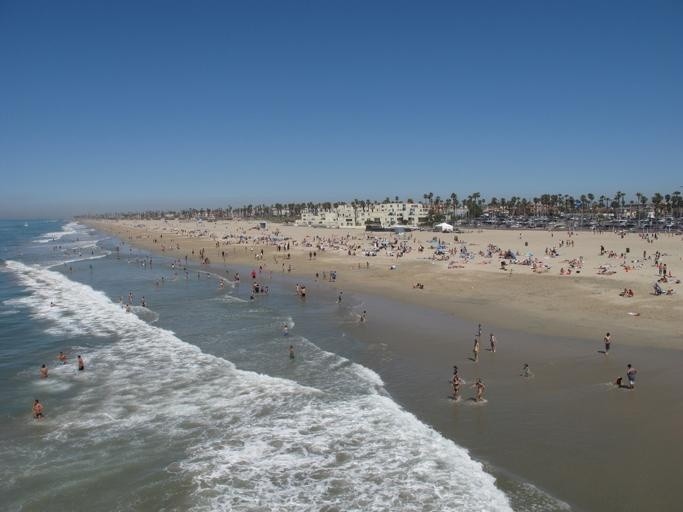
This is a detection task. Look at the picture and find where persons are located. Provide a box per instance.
[489,333,497,353]
[39,363,48,379]
[472,339,480,363]
[360,310,368,323]
[603,331,614,353]
[31,399,43,420]
[475,322,483,338]
[451,366,457,377]
[625,363,638,388]
[451,374,461,401]
[523,363,529,378]
[49,221,683,313]
[614,375,624,389]
[289,345,295,359]
[76,354,83,371]
[279,324,288,336]
[473,379,484,402]
[56,350,66,366]
